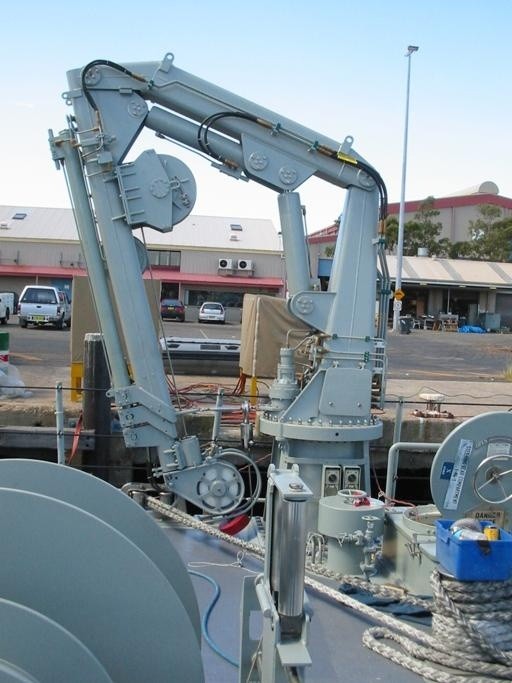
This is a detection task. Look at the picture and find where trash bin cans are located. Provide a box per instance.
[399,318,413,334]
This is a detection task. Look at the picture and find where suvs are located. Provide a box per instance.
[17,285,71,329]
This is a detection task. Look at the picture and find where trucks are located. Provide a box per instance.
[0,293,14,324]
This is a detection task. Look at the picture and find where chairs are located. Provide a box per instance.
[412,317,422,329]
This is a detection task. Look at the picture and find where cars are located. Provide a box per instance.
[160,299,185,322]
[198,301,226,324]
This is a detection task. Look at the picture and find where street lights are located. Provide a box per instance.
[392,45,419,331]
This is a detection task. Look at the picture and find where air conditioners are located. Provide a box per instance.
[218,258,254,273]
